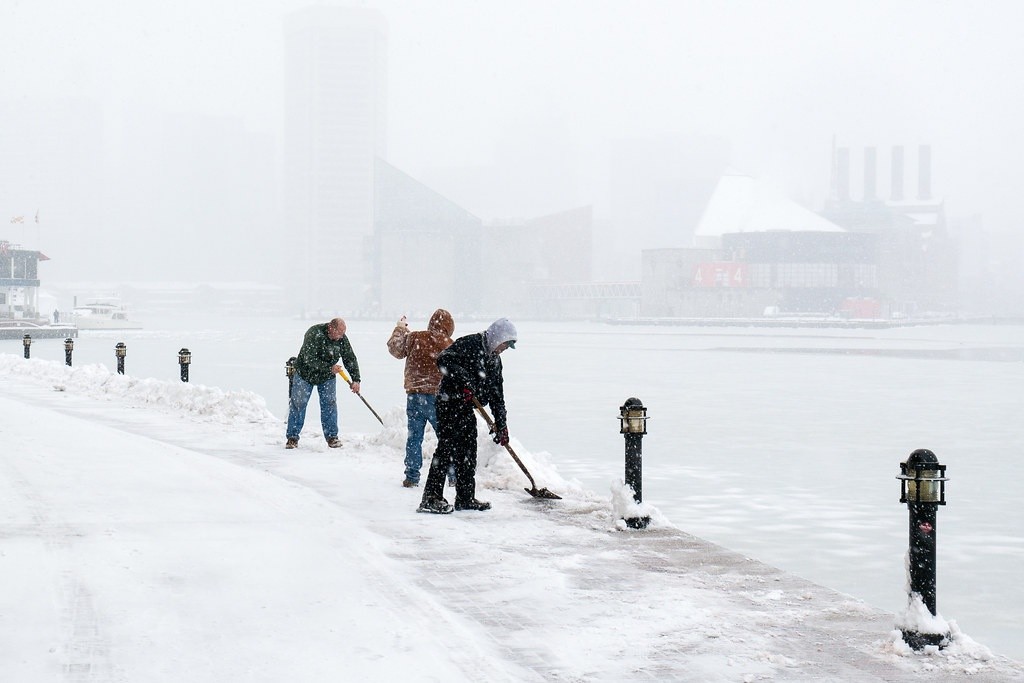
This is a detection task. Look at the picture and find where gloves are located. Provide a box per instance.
[489,424,509,445]
[458,373,474,403]
[397,315,408,328]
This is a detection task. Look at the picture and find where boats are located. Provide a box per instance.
[73,304,144,330]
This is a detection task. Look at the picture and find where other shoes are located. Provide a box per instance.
[286,437,298,449]
[326,437,343,448]
[403,480,417,488]
[455,498,491,510]
[420,493,454,514]
[448,478,456,486]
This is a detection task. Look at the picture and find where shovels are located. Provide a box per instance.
[472,394,562,499]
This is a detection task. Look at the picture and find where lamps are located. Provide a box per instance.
[64,339,74,351]
[23,335,32,345]
[285,357,298,376]
[896,449,949,506]
[114,343,127,357]
[617,398,650,436]
[177,349,190,365]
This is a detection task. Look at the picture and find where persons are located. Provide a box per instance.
[418,317,518,513]
[285,317,361,448]
[387,310,456,487]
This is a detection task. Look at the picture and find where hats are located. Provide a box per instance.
[503,340,516,349]
